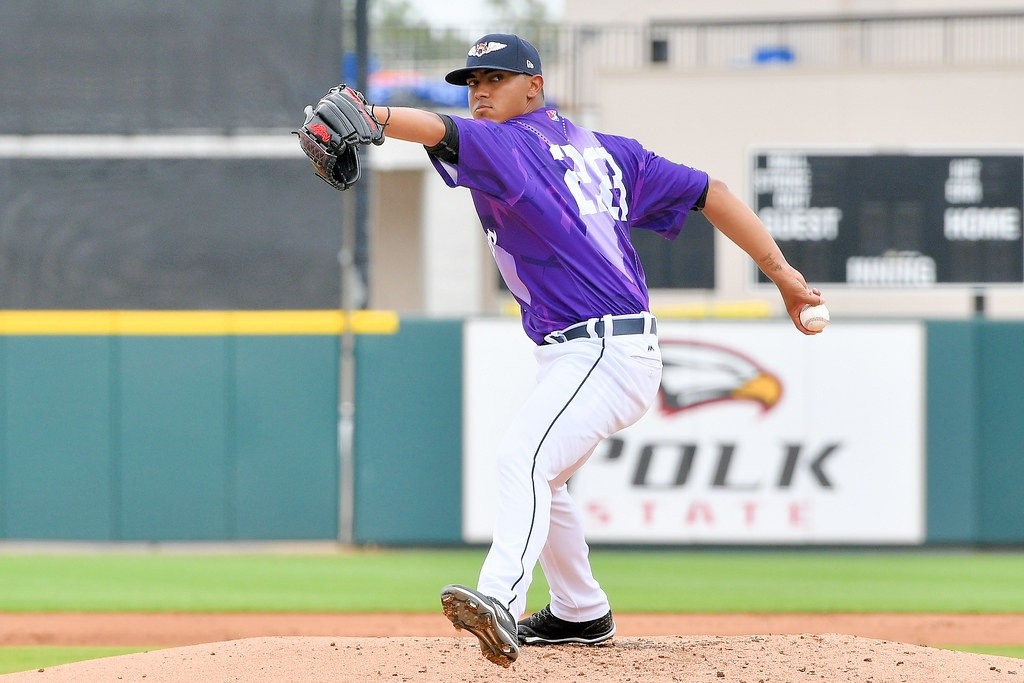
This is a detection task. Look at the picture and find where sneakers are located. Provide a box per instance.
[519,604,616,646]
[439,584,520,669]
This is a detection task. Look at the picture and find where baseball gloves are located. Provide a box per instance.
[289,82,387,190]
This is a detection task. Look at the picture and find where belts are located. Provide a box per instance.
[540,316,658,344]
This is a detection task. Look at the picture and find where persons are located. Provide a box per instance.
[297,34,824,668]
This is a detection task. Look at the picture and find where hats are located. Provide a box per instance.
[445,34,542,87]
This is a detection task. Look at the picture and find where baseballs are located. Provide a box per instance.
[799,300,831,332]
